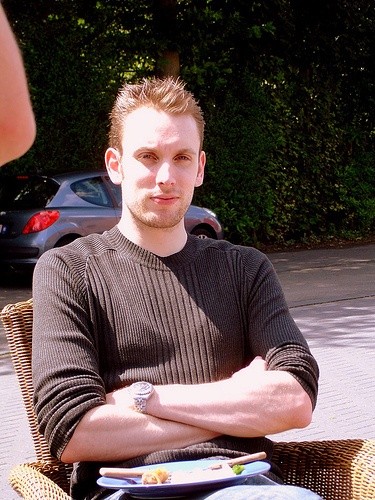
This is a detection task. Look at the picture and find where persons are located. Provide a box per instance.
[0,4,37,167]
[31,76,322,500]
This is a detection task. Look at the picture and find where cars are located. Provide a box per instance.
[0,168,225,279]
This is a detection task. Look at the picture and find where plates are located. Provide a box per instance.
[96,460,270,495]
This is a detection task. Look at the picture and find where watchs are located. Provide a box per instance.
[129,381,155,415]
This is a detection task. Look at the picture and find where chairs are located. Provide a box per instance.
[0,298,375,500]
